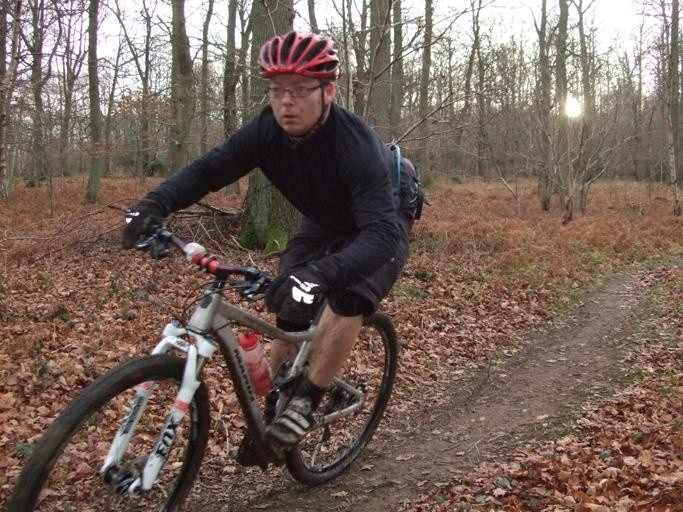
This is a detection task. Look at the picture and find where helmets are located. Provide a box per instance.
[258,31,340,82]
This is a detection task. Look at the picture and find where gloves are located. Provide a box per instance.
[121,192,173,249]
[267,258,329,332]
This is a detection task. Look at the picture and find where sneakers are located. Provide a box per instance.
[263,394,317,449]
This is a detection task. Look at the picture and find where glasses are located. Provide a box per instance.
[264,85,323,97]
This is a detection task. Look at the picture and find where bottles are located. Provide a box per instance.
[237,330,274,397]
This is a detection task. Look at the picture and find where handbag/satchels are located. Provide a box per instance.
[391,145,424,221]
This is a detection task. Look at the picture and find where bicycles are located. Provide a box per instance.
[9,204,397,512]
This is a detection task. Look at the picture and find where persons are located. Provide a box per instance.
[123,31,410,466]
